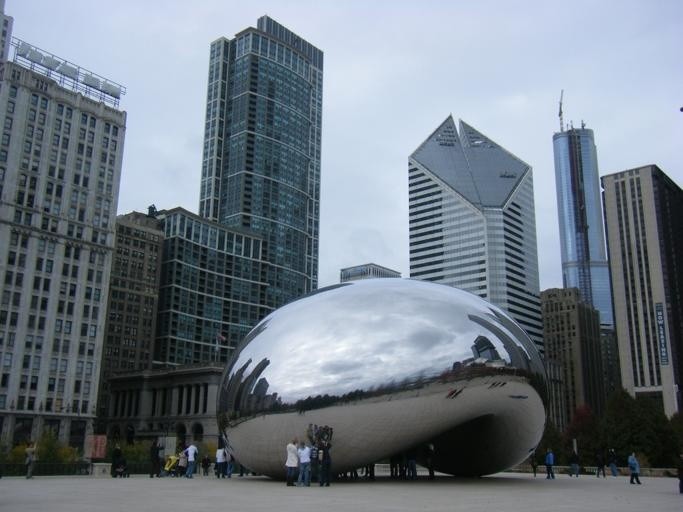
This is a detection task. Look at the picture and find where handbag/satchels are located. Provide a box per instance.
[629,466,636,471]
[25,458,31,465]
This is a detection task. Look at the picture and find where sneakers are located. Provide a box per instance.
[112,475,130,478]
[287,483,329,487]
[150,474,193,479]
[216,474,231,478]
[630,481,641,484]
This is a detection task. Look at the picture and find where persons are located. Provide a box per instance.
[596,448,618,478]
[176,442,199,478]
[628,452,641,484]
[569,450,580,478]
[339,464,375,480]
[390,444,417,481]
[216,443,232,478]
[529,454,538,479]
[677,453,683,494]
[112,459,129,478]
[202,455,210,475]
[150,440,164,478]
[25,440,38,478]
[284,441,332,486]
[238,465,256,476]
[424,443,435,479]
[545,448,555,479]
